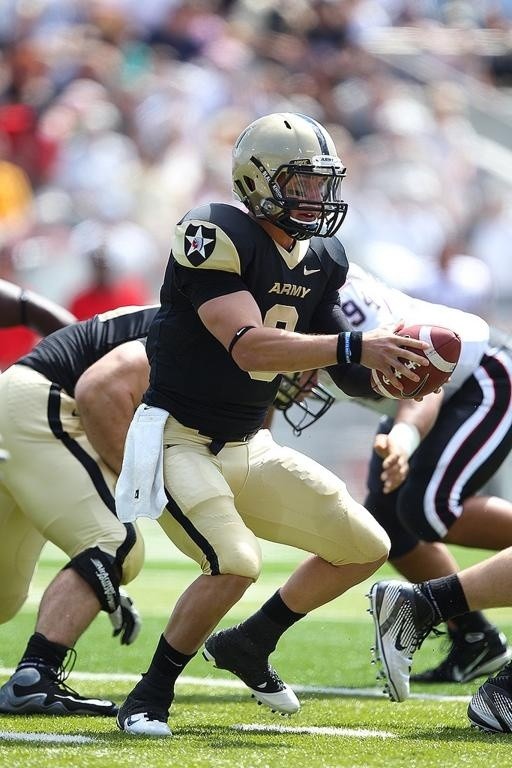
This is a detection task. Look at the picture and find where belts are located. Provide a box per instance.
[201,431,259,442]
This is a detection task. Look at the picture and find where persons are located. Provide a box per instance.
[116,111,396,739]
[0,290,175,716]
[1,0,511,346]
[273,253,510,683]
[366,545,512,739]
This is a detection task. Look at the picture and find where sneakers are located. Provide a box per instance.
[1,658,116,715]
[368,581,512,741]
[113,692,172,739]
[202,626,300,716]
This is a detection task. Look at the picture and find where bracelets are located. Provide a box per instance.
[384,421,420,458]
[336,329,363,364]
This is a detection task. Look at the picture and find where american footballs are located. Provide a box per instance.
[371,324,461,400]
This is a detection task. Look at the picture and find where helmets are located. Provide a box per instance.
[231,111,347,237]
[274,365,339,436]
